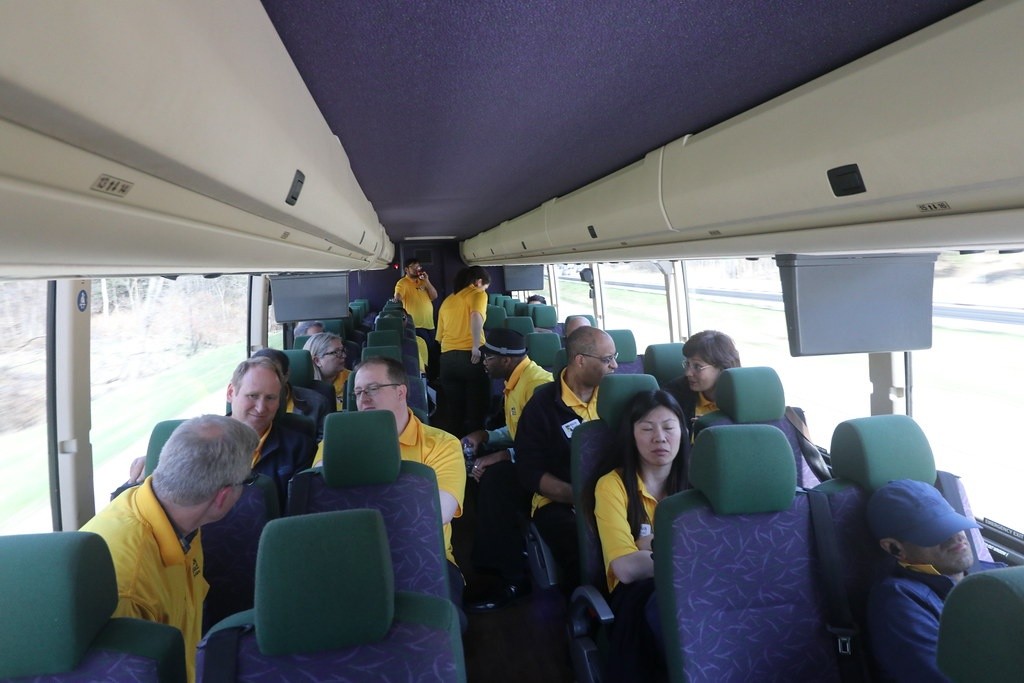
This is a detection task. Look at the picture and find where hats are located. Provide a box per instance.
[477,327,527,357]
[866,479,983,547]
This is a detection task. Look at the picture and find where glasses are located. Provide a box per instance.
[407,265,422,270]
[351,383,401,400]
[682,359,715,373]
[322,347,347,358]
[219,471,259,490]
[575,351,619,366]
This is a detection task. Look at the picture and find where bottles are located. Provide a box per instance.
[463,439,474,472]
[417,268,424,279]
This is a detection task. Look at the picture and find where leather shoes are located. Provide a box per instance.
[470,582,531,614]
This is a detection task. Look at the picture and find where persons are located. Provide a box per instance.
[79,414,260,683]
[593,390,693,683]
[129,356,318,519]
[294,307,428,379]
[252,348,333,443]
[679,331,741,443]
[528,295,546,304]
[303,332,351,411]
[435,266,492,439]
[514,327,618,559]
[311,357,467,609]
[567,317,591,338]
[864,479,1009,683]
[460,328,555,613]
[390,259,437,380]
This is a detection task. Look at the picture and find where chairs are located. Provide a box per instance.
[0,293,1024,683]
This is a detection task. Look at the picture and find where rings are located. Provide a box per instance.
[475,466,477,468]
